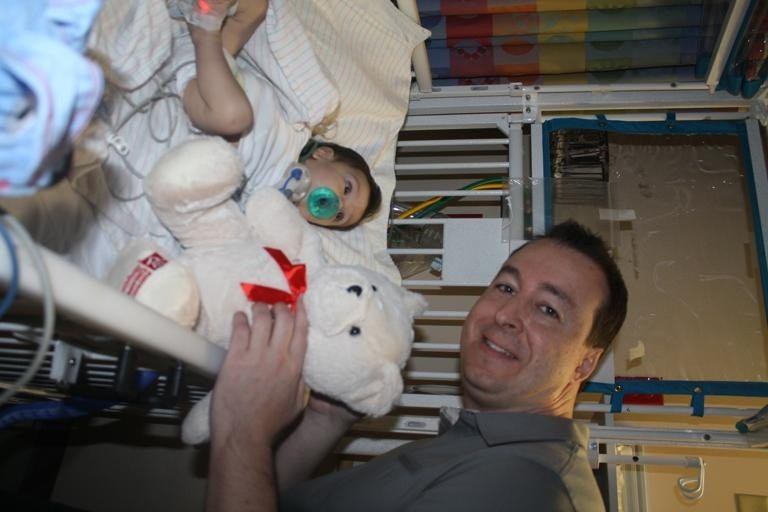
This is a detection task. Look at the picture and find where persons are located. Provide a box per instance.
[206,216,629,510]
[174,1,384,235]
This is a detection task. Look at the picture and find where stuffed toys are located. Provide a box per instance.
[142,132,429,447]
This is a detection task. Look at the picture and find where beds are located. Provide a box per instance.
[0,0,434,411]
